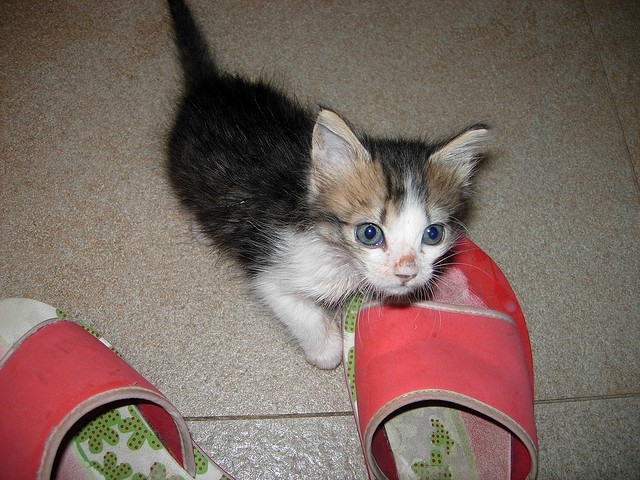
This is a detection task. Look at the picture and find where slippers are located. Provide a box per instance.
[338,238,536,476]
[0,297,237,480]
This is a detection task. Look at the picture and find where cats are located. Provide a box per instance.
[162,0,493,370]
